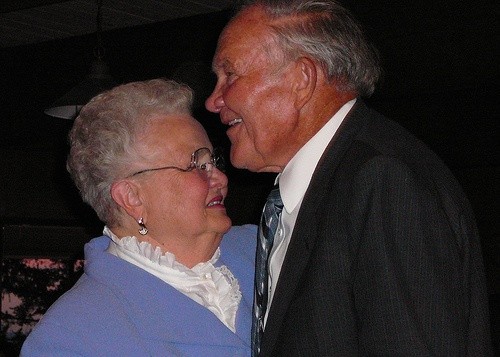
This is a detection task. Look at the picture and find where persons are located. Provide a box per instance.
[205,0,493,357]
[17,78,258,357]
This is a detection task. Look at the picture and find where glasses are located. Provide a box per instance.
[124,145,226,180]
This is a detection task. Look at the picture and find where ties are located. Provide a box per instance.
[251,183,284,357]
